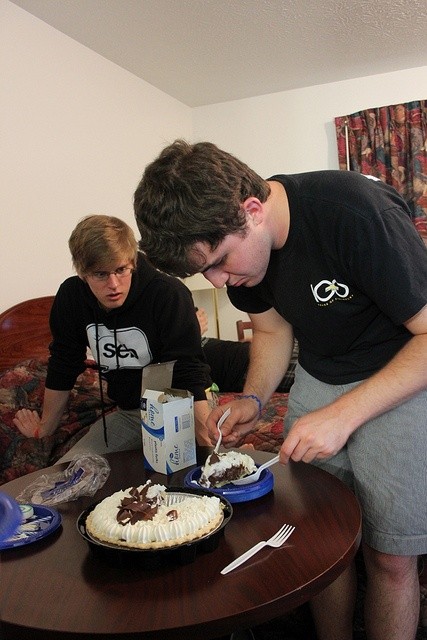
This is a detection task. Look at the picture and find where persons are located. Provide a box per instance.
[132,139,427,639]
[14,215,255,466]
[196,309,252,393]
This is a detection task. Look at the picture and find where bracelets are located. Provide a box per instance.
[241,395,261,417]
[35,427,40,439]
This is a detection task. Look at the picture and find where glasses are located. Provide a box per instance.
[87,266,134,281]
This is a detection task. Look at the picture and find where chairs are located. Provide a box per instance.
[236,318,254,341]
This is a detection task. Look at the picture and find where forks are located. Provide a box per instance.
[203,409,234,464]
[220,524,295,575]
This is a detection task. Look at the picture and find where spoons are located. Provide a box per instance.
[231,454,281,486]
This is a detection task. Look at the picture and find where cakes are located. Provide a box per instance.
[198,450,257,487]
[86,480,224,550]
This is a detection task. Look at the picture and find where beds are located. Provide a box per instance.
[1,295,426,640]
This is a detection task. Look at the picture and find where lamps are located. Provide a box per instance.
[177,272,226,340]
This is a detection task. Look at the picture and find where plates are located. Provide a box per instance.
[0,504,61,550]
[76,484,233,571]
[184,461,274,504]
